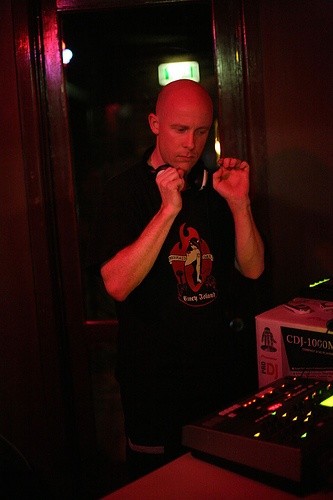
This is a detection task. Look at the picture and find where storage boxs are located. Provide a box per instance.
[255,297,333,389]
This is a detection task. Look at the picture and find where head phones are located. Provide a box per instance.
[144,145,208,191]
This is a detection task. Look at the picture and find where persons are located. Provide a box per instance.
[93,80,264,483]
